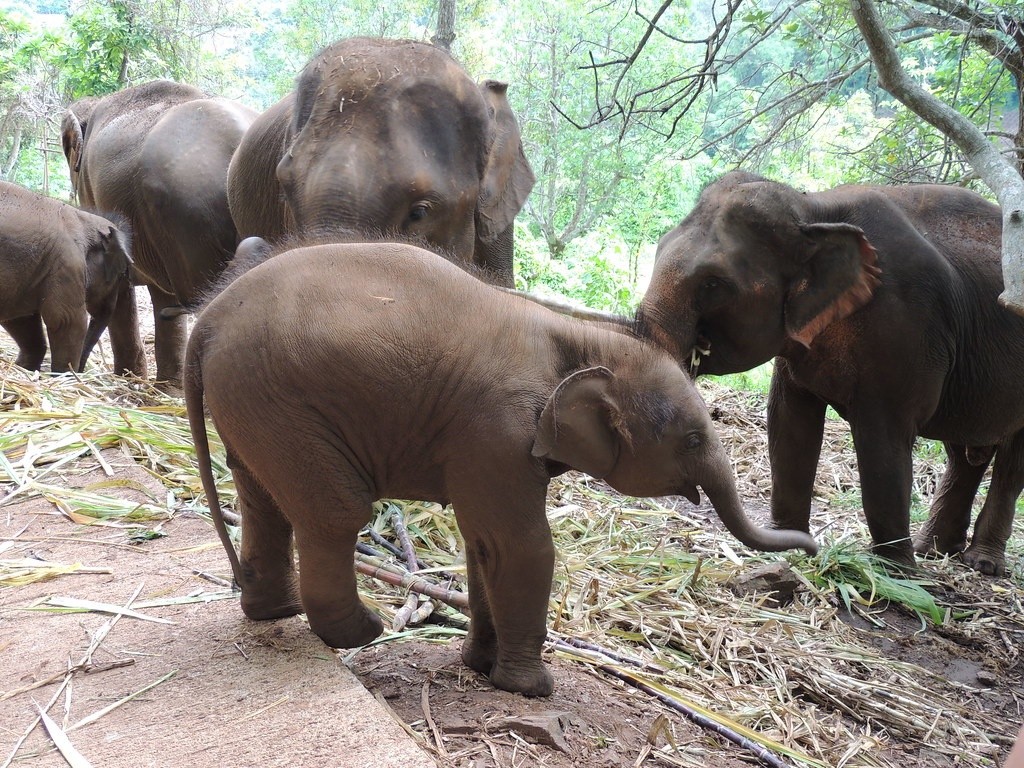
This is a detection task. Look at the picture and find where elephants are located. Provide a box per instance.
[0,35,1024,695]
